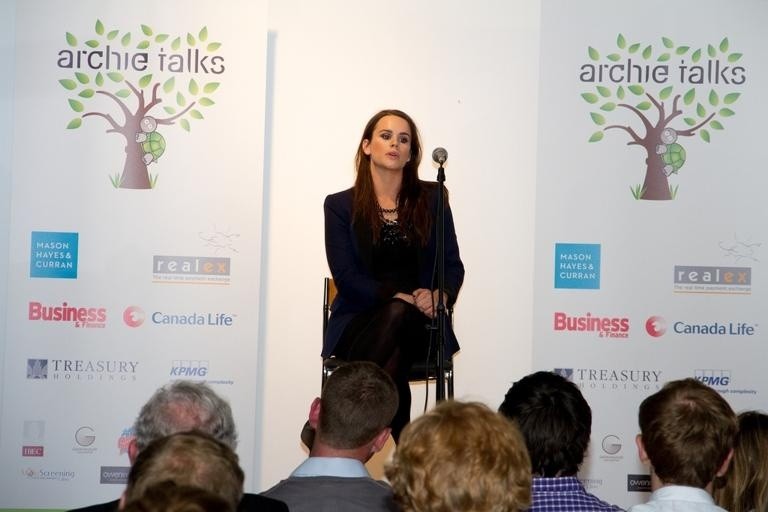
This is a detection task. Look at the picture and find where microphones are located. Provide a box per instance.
[432,147,448,165]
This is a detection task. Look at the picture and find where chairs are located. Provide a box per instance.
[318,277,456,415]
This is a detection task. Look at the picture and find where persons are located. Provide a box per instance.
[387,400,533,511]
[253,360,403,510]
[118,431,244,511]
[629,377,740,510]
[711,408,768,510]
[319,110,462,430]
[65,381,288,510]
[498,370,625,511]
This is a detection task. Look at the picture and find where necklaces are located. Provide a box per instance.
[377,198,400,213]
